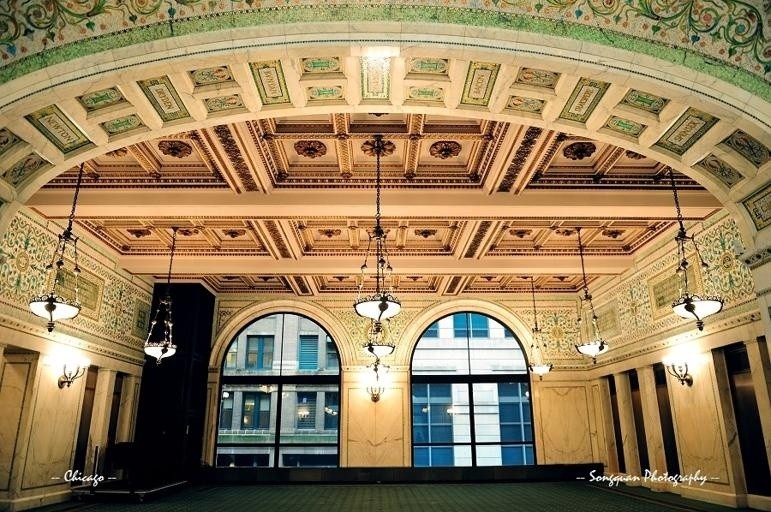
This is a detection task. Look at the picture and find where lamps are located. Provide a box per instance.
[143,230,178,364]
[528,277,553,381]
[29,162,84,332]
[574,228,609,365]
[662,356,693,386]
[353,137,402,402]
[668,167,724,331]
[58,357,90,388]
